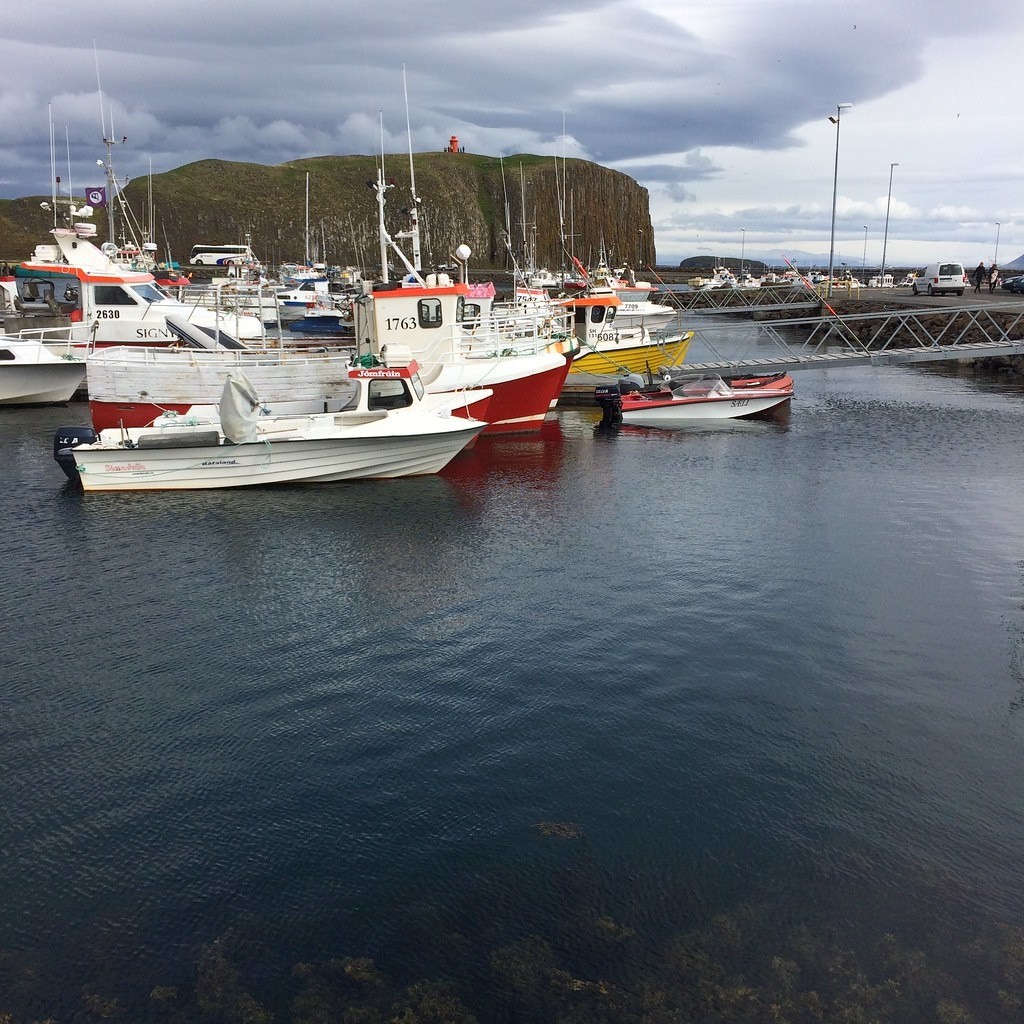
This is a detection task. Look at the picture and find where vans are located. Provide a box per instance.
[912,261,972,296]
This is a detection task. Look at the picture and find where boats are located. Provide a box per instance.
[49,358,492,494]
[1,48,895,452]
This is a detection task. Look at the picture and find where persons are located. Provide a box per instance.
[916,270,924,277]
[460,146,464,153]
[988,264,999,294]
[970,261,988,294]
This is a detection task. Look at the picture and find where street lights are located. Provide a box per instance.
[880,163,900,286]
[826,101,852,297]
[862,225,867,279]
[741,228,746,283]
[993,222,1001,263]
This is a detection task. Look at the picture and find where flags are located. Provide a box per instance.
[86,187,106,208]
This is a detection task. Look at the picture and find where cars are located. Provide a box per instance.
[1002,275,1024,294]
